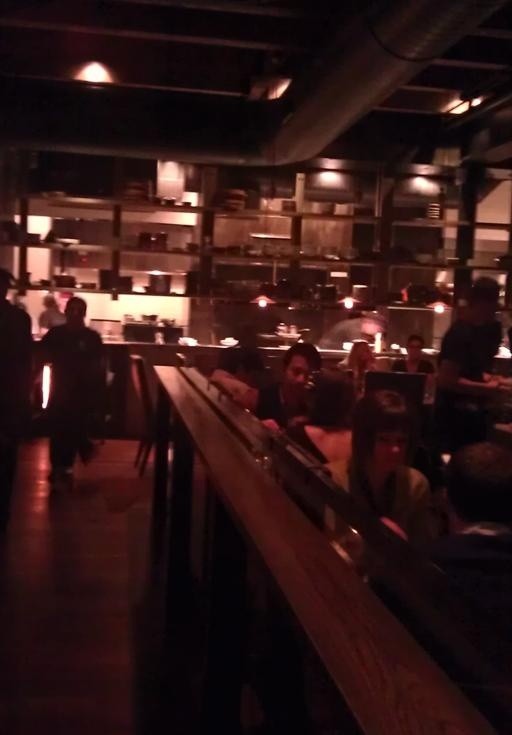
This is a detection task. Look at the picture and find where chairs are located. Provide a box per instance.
[365,369,428,429]
[435,384,489,440]
[129,354,181,475]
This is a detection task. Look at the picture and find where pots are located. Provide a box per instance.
[139,232,167,251]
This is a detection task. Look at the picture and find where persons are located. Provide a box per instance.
[437,275,502,374]
[221,327,512,666]
[38,294,67,329]
[39,295,107,487]
[1,268,37,529]
[319,310,376,350]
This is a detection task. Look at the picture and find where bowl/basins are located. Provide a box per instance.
[162,318,176,326]
[415,253,433,265]
[142,314,158,322]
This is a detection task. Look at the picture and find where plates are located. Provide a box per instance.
[275,332,301,339]
[220,340,240,346]
[77,280,97,290]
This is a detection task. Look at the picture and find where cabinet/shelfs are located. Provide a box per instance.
[0,151,512,305]
